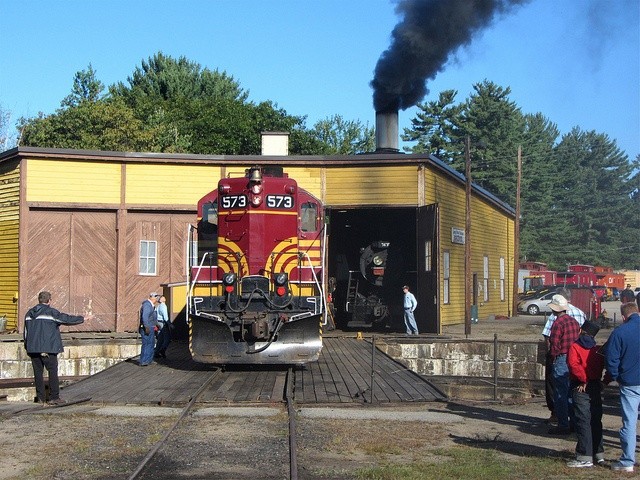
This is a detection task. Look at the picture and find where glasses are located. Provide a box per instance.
[627,286,631,287]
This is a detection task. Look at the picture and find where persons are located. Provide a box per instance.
[566,320,605,468]
[156,295,174,360]
[604,302,640,473]
[139,292,159,367]
[620,284,635,301]
[23,291,95,404]
[542,298,588,425]
[400,285,419,337]
[548,294,581,433]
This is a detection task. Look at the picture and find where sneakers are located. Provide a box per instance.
[544,416,559,423]
[566,460,594,468]
[572,427,578,433]
[548,428,571,435]
[610,462,634,472]
[595,459,604,464]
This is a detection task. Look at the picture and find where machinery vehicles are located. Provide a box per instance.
[517,275,547,297]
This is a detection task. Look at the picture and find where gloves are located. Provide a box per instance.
[146,327,150,336]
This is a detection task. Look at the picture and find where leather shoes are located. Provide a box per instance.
[48,398,66,405]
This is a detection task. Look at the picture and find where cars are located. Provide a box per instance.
[516,286,570,316]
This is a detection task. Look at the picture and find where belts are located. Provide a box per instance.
[403,308,410,309]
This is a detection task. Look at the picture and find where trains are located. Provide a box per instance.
[185,163,329,368]
[329,239,417,332]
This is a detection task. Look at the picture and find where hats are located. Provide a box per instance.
[149,292,161,298]
[402,285,410,289]
[581,320,600,336]
[546,294,571,312]
